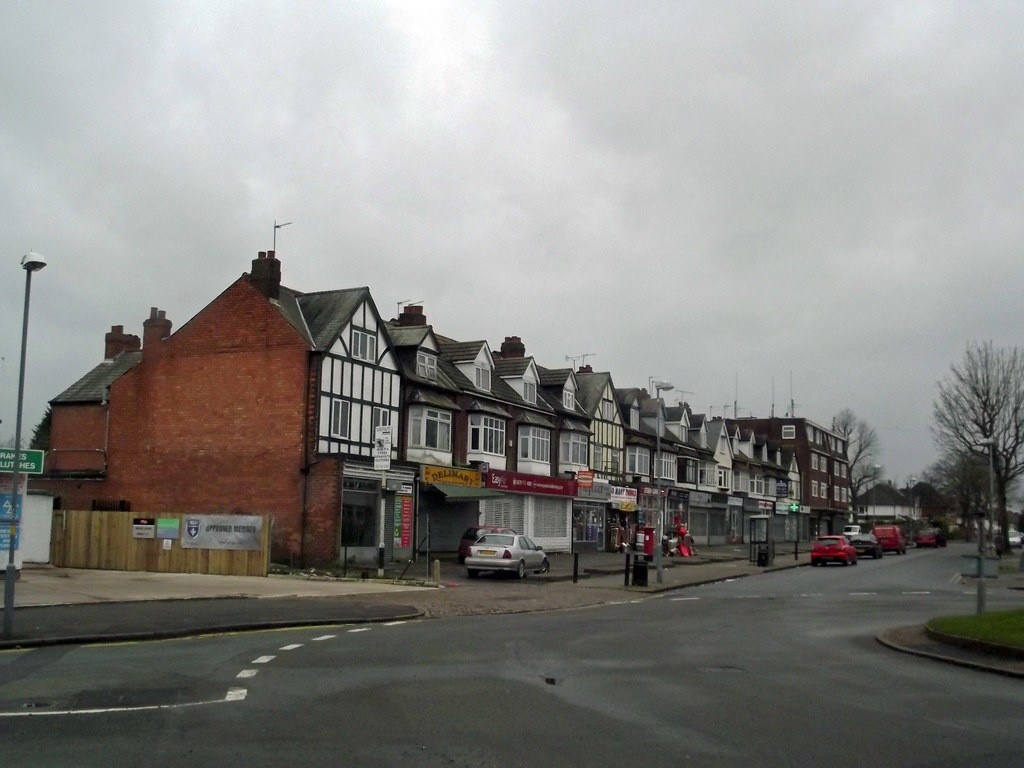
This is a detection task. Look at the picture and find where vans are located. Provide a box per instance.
[874,524,909,557]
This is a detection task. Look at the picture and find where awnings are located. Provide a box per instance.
[430,483,505,504]
[810,507,863,517]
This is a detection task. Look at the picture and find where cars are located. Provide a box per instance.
[456,526,518,567]
[848,532,881,559]
[917,529,936,547]
[1008,529,1022,549]
[810,534,858,567]
[465,533,551,581]
[930,527,946,545]
[841,524,863,539]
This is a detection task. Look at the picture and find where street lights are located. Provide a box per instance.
[1,251,47,643]
[979,436,998,555]
[654,380,675,584]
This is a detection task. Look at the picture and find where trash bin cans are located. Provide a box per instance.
[756,544,769,567]
[631,552,649,587]
